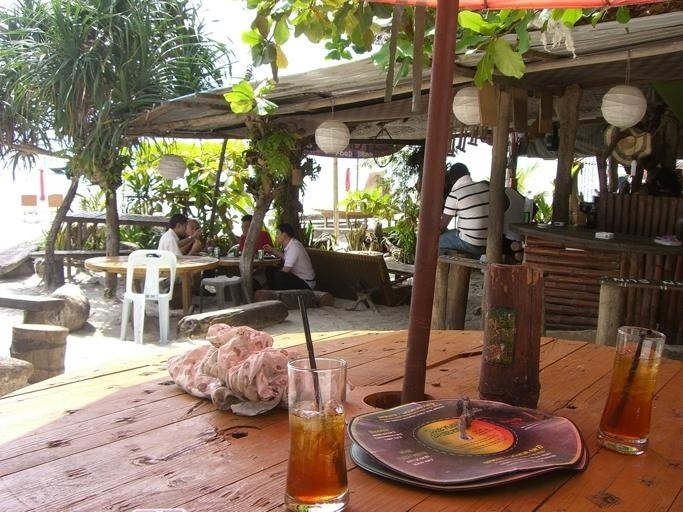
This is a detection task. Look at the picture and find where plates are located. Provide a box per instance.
[263,256,279,258]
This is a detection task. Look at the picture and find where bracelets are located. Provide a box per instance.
[188,236,192,240]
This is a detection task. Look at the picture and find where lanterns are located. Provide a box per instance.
[158,154,186,180]
[601,84,647,128]
[453,87,481,125]
[315,118,350,154]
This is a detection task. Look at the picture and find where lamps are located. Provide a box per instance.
[315,98,350,154]
[158,137,185,180]
[601,49,647,130]
[452,69,482,126]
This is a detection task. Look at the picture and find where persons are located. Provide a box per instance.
[238,215,275,291]
[480,180,526,253]
[180,219,200,256]
[263,224,317,291]
[438,163,510,253]
[158,214,202,286]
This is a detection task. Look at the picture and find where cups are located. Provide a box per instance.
[208,246,220,260]
[283,357,351,511]
[257,249,264,261]
[595,324,665,458]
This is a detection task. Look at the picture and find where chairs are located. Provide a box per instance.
[120,249,177,346]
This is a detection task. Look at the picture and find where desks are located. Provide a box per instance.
[387,264,415,282]
[65,210,169,278]
[321,211,372,227]
[0,294,65,324]
[0,329,683,512]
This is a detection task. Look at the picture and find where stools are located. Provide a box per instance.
[10,324,69,384]
[200,275,251,313]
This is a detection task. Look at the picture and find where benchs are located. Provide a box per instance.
[178,300,289,339]
[255,290,333,309]
[28,250,135,285]
[431,250,550,336]
[304,247,412,306]
[595,275,683,346]
